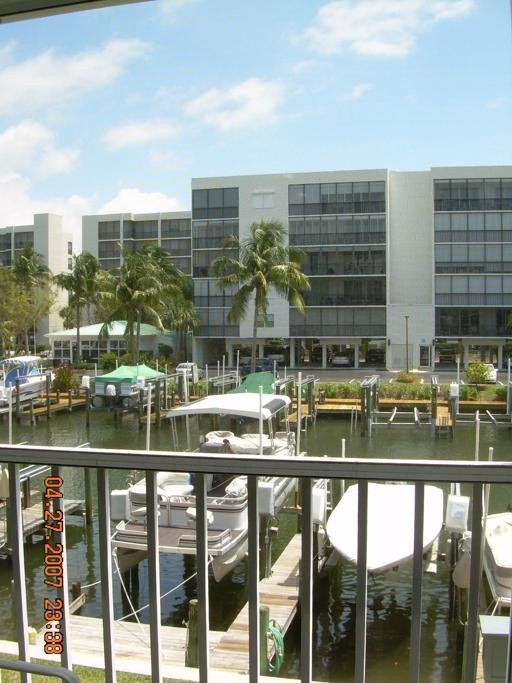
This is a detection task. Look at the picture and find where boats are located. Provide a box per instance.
[110,391,308,579]
[0,356,55,407]
[326,480,444,577]
[483,511,512,605]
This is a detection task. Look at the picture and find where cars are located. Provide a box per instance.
[310,347,330,364]
[366,348,385,364]
[267,347,290,365]
[241,358,280,373]
[331,351,354,366]
[176,362,204,381]
[479,363,499,383]
[439,350,457,363]
